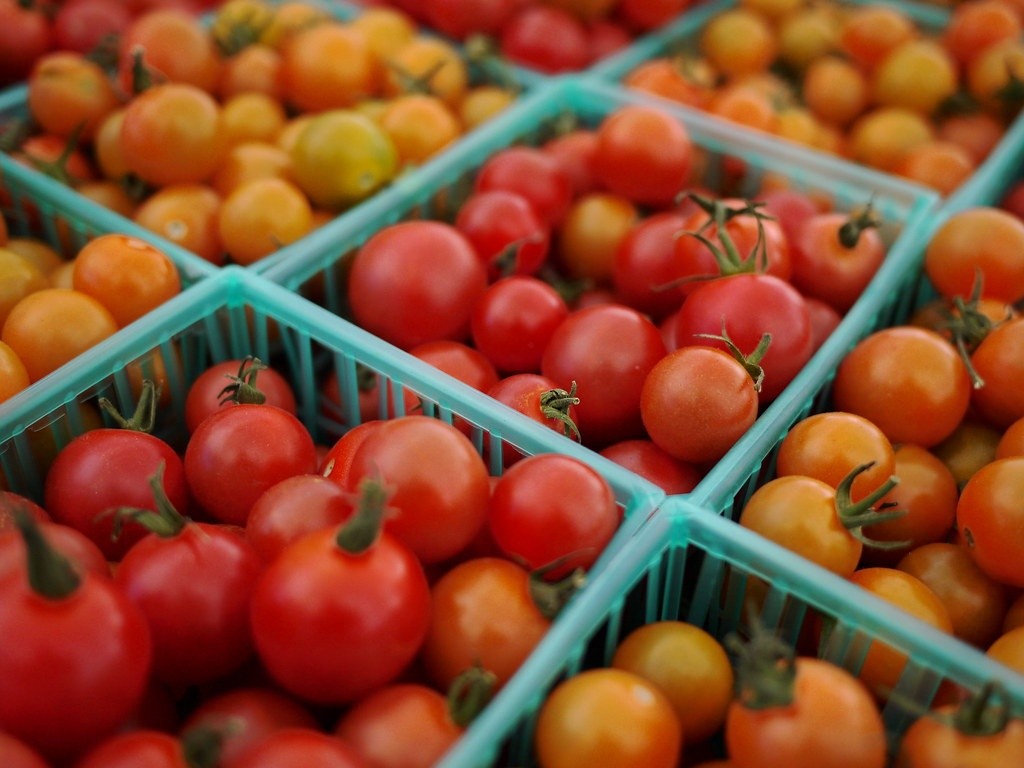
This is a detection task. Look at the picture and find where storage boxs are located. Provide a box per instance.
[676,210,1024,695]
[0,264,666,768]
[0,0,556,276]
[0,156,224,494]
[259,74,944,499]
[578,0,1024,208]
[441,497,1024,768]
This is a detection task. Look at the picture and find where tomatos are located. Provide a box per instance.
[0,0,1024,768]
